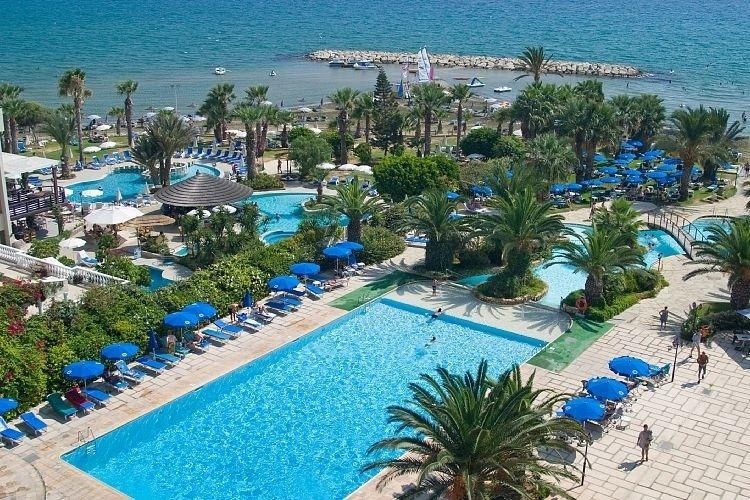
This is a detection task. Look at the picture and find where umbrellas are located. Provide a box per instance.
[323,245,352,275]
[64,359,105,400]
[82,146,102,154]
[495,170,513,180]
[87,115,102,121]
[244,289,253,317]
[182,301,218,333]
[291,262,320,294]
[100,343,142,380]
[96,125,111,135]
[148,328,158,361]
[82,202,143,241]
[268,275,301,298]
[316,162,337,175]
[164,311,199,343]
[587,376,629,404]
[335,240,364,265]
[737,308,750,320]
[471,184,492,199]
[467,151,486,161]
[0,398,20,416]
[563,395,607,440]
[164,106,176,112]
[99,142,117,149]
[445,191,460,204]
[355,165,374,183]
[338,164,359,176]
[547,137,732,207]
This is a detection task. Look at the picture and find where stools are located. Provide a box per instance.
[620,417,631,429]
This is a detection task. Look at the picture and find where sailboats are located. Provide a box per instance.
[417,45,437,82]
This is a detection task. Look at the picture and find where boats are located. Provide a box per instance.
[215,67,225,75]
[328,58,377,70]
[494,86,512,92]
[453,77,486,87]
[270,71,277,76]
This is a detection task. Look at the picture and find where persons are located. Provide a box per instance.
[83,224,117,246]
[343,263,354,270]
[744,116,748,122]
[637,424,654,463]
[741,110,745,120]
[159,231,165,242]
[473,194,488,203]
[658,306,669,332]
[138,233,147,242]
[71,381,87,403]
[424,336,445,348]
[651,371,661,382]
[232,165,236,177]
[430,276,439,297]
[426,308,443,327]
[624,379,639,390]
[167,341,176,356]
[253,298,270,316]
[323,275,350,287]
[606,402,616,412]
[657,253,663,265]
[696,351,709,384]
[575,294,589,317]
[192,334,205,344]
[688,328,703,359]
[744,161,750,177]
[644,232,656,251]
[277,158,283,173]
[227,303,243,321]
[103,365,122,385]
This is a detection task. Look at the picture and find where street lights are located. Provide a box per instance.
[671,337,680,382]
[581,433,593,486]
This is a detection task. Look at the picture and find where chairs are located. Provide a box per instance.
[20,412,48,435]
[175,345,190,356]
[407,235,429,247]
[201,328,231,344]
[28,146,248,186]
[137,355,167,375]
[236,313,263,331]
[463,203,486,216]
[553,181,725,209]
[114,360,145,384]
[267,275,328,315]
[105,375,129,392]
[18,142,26,153]
[183,330,209,353]
[738,348,750,364]
[649,363,672,383]
[150,348,181,368]
[250,309,273,322]
[82,385,109,406]
[330,176,378,196]
[0,416,26,447]
[65,388,95,415]
[214,319,243,338]
[81,193,143,213]
[77,246,189,268]
[47,392,78,420]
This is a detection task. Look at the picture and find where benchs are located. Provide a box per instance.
[587,420,608,437]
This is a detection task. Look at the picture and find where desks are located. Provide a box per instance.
[735,334,750,352]
